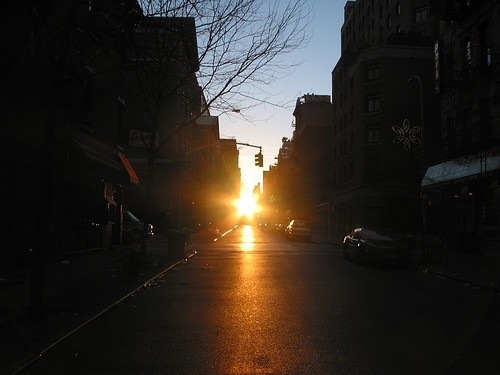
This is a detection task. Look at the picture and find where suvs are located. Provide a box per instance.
[289,220,312,243]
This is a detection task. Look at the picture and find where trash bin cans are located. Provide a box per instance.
[169,225,192,259]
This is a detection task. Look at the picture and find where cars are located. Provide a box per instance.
[273,223,289,236]
[342,227,410,269]
[114,211,154,242]
[259,222,267,228]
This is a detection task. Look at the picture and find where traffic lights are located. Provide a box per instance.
[255,152,263,167]
